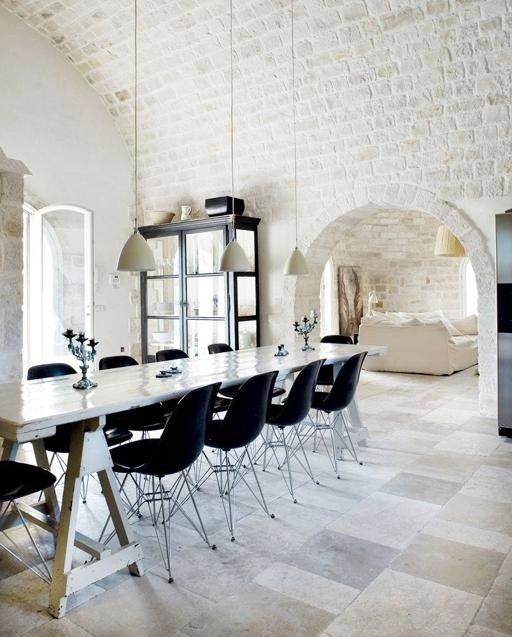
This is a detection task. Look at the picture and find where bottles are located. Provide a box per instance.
[211,293,221,317]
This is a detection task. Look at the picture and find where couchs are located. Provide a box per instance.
[359,309,480,376]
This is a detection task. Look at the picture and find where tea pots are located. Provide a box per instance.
[180,205,192,220]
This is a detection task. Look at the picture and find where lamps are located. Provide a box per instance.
[366,289,378,317]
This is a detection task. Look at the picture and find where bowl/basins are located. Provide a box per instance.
[149,211,175,224]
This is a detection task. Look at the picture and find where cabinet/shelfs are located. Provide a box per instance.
[134,213,263,365]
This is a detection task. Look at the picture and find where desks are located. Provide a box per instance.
[0,340,390,619]
